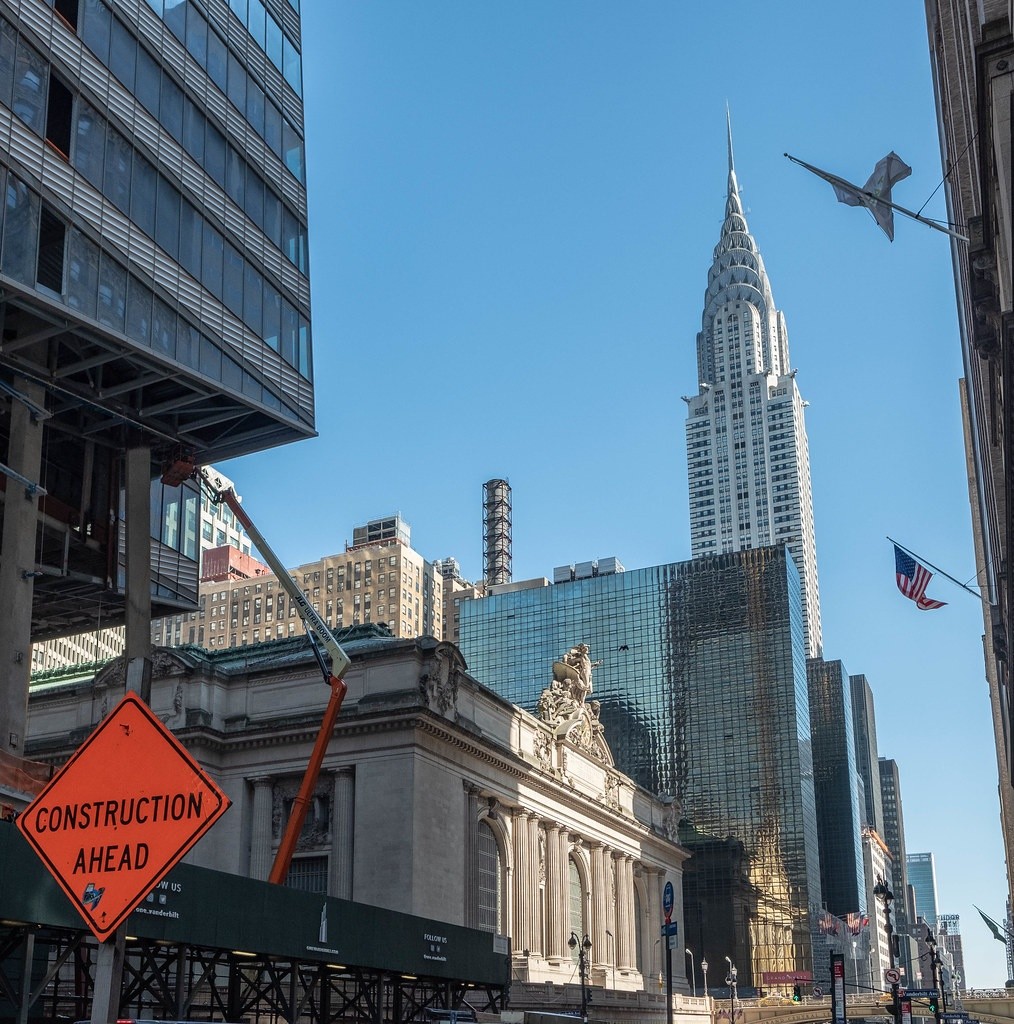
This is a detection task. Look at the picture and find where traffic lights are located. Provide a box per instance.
[924,927,949,1024]
[929,1005,937,1013]
[884,1004,894,1015]
[792,985,802,1002]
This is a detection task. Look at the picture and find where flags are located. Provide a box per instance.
[893,543,949,611]
[788,151,913,245]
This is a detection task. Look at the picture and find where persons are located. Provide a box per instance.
[539,642,605,735]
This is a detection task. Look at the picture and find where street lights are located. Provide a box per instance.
[567,931,597,1024]
[701,957,710,996]
[851,941,860,998]
[725,957,734,999]
[652,938,663,995]
[605,930,616,989]
[868,948,879,994]
[684,949,697,998]
[873,874,906,1024]
